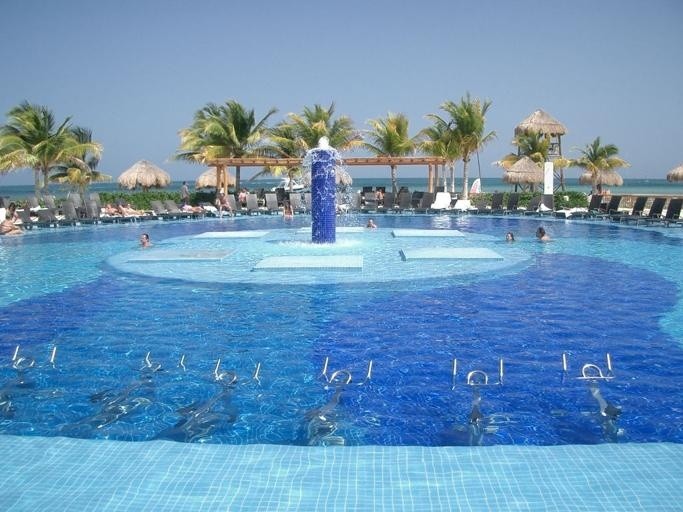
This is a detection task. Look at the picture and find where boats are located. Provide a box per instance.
[270,174,306,191]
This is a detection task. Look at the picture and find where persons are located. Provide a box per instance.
[377,189,385,200]
[282,201,293,221]
[6,202,18,223]
[183,203,202,214]
[366,219,377,228]
[0,212,23,236]
[537,226,550,241]
[125,203,146,215]
[104,204,125,216]
[140,233,151,249]
[238,188,248,205]
[505,231,515,242]
[218,193,232,216]
[180,180,190,203]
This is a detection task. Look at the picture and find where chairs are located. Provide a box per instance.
[0,182,682,234]
